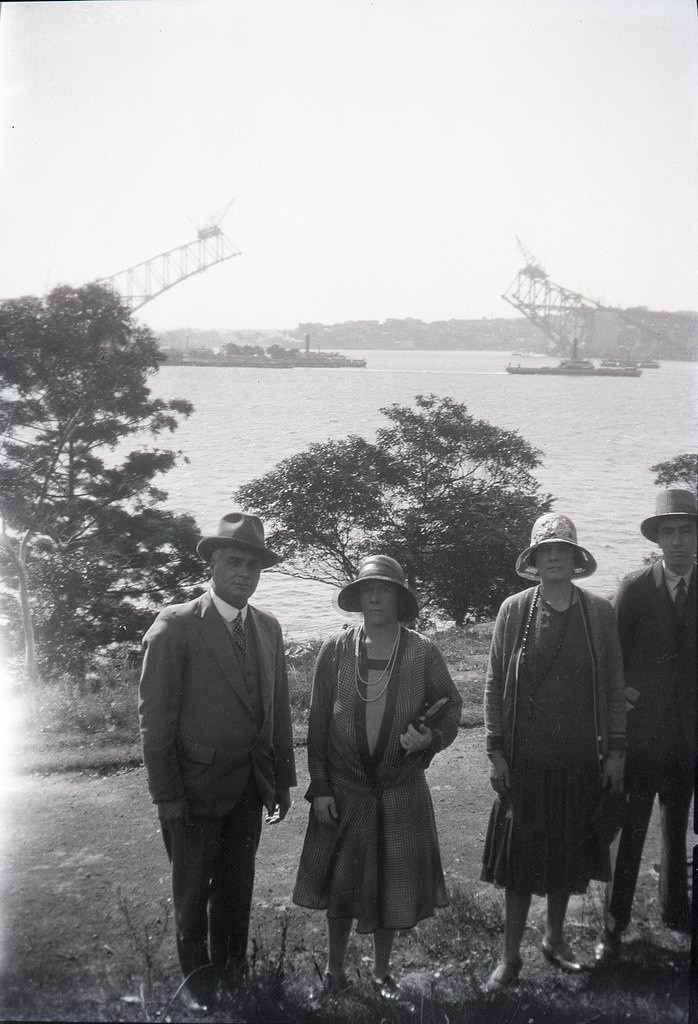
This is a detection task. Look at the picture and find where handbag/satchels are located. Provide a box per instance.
[592,788,628,847]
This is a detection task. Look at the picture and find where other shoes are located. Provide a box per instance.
[484,958,523,992]
[323,973,375,1014]
[371,975,420,1014]
[184,977,257,1016]
[542,937,582,972]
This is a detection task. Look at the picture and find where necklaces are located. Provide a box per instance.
[355,620,401,702]
[522,583,574,694]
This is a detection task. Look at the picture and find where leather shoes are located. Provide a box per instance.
[595,925,620,961]
[662,913,693,933]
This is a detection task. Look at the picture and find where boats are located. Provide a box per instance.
[506,337,643,377]
[602,347,659,368]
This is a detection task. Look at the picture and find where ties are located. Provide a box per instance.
[674,578,687,635]
[233,611,246,657]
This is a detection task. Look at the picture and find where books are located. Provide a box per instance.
[402,697,450,758]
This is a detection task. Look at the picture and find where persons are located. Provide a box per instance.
[480,513,626,992]
[291,555,463,998]
[601,490,698,952]
[138,514,298,1015]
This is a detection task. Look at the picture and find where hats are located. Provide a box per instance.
[338,555,418,621]
[640,489,698,543]
[196,513,279,569]
[515,513,597,581]
